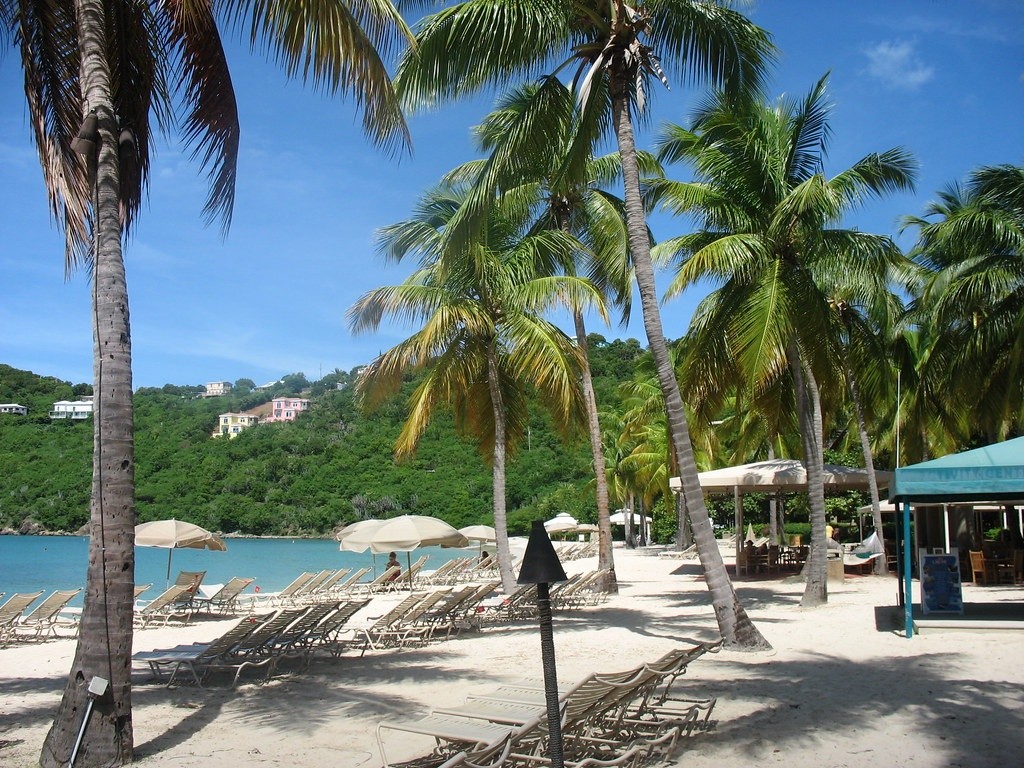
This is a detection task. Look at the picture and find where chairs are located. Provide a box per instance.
[969,549,996,587]
[738,542,809,579]
[997,549,1023,586]
[0,527,726,768]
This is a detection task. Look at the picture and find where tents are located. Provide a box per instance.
[669,458,915,577]
[889,436,1024,638]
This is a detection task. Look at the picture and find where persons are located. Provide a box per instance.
[478,551,493,568]
[378,552,401,592]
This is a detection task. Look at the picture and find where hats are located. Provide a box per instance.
[746,540,754,544]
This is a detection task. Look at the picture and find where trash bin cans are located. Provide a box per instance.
[827,549,845,581]
[722,531,730,539]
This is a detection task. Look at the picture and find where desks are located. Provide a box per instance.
[983,557,1011,585]
[751,555,769,574]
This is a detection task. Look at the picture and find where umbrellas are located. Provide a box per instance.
[135,509,652,594]
